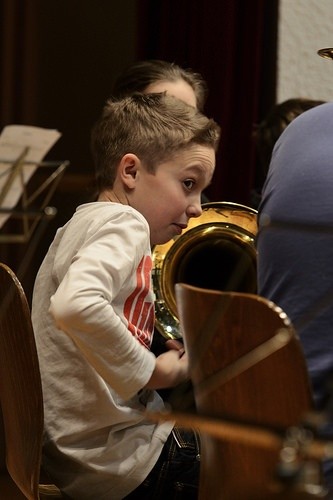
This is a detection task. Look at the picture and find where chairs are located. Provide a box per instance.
[175,281,333,500]
[0,262,62,500]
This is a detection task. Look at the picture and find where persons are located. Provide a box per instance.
[31,62,219,500]
[257,103,333,412]
